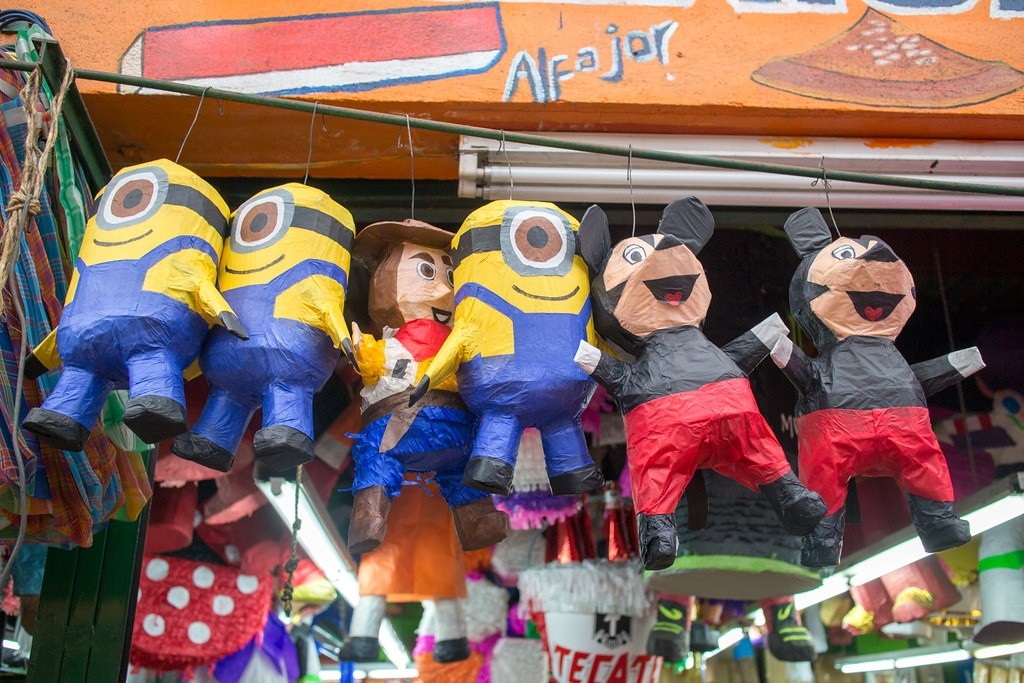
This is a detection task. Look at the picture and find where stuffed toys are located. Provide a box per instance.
[412,526,548,683]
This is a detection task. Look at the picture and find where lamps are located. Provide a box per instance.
[457,127,1024,214]
[247,455,412,669]
[676,470,1024,683]
